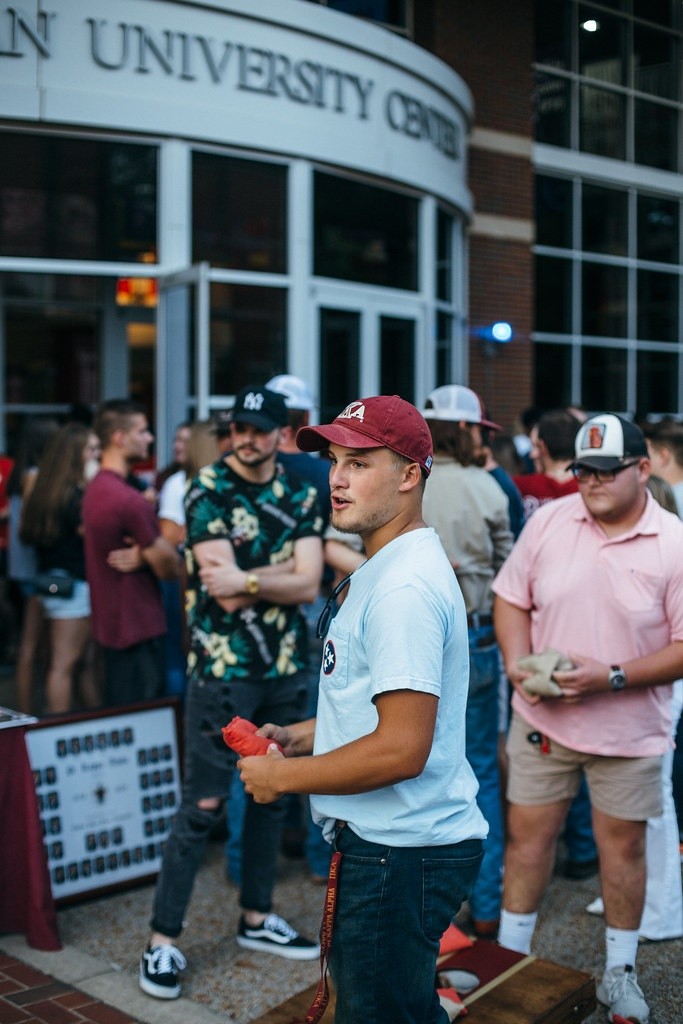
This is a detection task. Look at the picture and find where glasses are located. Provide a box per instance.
[571,459,639,483]
[233,421,267,435]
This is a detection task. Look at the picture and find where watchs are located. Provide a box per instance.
[609,662,627,692]
[245,568,260,596]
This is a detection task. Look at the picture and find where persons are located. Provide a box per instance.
[234,395,489,1023]
[0,374,683,941]
[489,414,683,1024]
[139,385,321,999]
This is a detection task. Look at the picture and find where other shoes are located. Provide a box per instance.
[586,895,604,915]
[308,868,331,887]
[567,858,600,879]
[454,911,499,944]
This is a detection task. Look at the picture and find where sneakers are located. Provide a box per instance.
[138,940,187,999]
[236,913,319,960]
[596,965,650,1024]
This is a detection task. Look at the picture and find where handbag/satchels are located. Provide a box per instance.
[35,576,73,599]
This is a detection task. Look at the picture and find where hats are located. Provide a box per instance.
[296,395,434,480]
[421,385,504,430]
[265,375,316,411]
[563,412,651,473]
[231,385,289,434]
